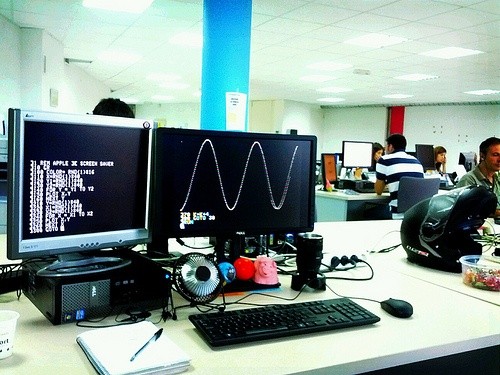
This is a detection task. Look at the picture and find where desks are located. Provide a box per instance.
[314,184,448,222]
[0,219,500,375]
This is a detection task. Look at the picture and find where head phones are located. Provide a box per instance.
[331,255,357,272]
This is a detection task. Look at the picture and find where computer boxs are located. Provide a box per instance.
[338,179,375,190]
[18,250,172,324]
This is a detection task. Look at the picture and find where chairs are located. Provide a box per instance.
[364,177,441,220]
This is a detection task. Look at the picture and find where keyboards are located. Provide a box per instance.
[188,296,379,348]
[356,188,390,193]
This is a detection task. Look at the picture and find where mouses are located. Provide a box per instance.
[381,300,414,318]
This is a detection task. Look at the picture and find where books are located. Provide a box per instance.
[77,321,191,375]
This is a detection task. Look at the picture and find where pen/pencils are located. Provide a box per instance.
[130,328,163,361]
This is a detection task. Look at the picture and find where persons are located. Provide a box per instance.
[457,137,500,216]
[375,133,424,220]
[423,146,446,174]
[368,143,385,171]
[93,98,135,118]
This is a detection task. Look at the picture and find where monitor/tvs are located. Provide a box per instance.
[415,144,437,170]
[152,127,317,259]
[342,141,372,168]
[459,152,477,170]
[321,153,344,183]
[8,108,152,275]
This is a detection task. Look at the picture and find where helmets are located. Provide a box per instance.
[401,185,498,274]
